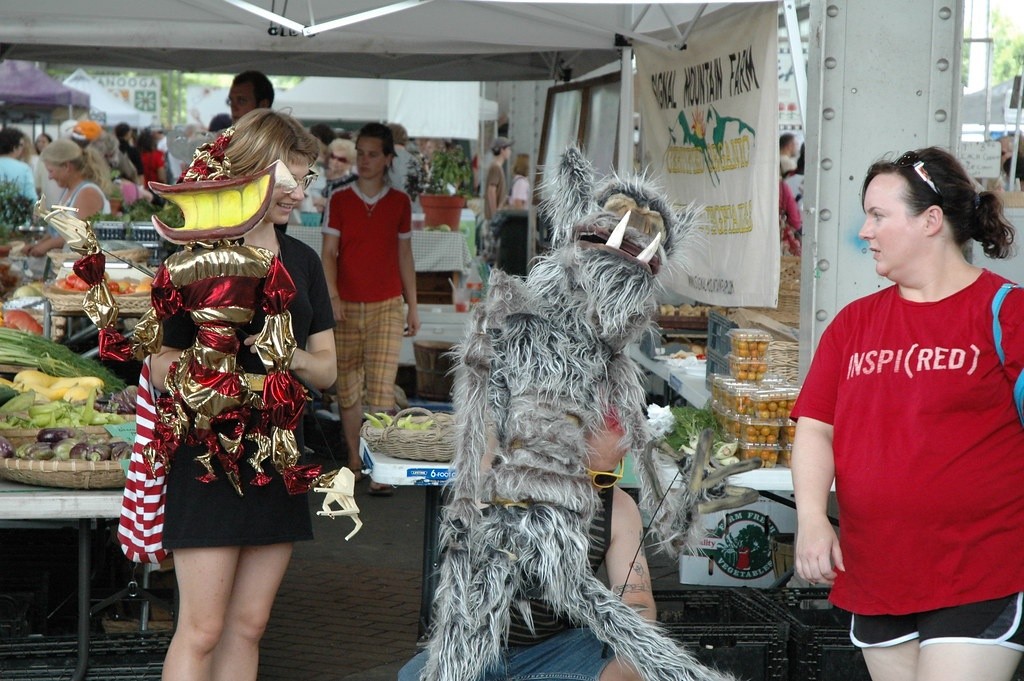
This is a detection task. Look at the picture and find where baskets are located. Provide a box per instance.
[47,247,150,269]
[360,407,459,461]
[0,422,136,488]
[43,283,153,313]
[768,341,798,385]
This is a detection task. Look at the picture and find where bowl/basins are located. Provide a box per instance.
[708,326,802,468]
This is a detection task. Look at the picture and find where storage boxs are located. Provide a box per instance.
[131,221,160,241]
[92,221,126,240]
[678,490,831,588]
[142,243,162,259]
[741,585,872,681]
[704,310,802,468]
[652,590,790,681]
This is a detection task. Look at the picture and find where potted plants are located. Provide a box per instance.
[0,179,21,258]
[404,143,473,232]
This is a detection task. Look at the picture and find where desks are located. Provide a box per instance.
[668,372,712,410]
[357,437,456,650]
[44,300,147,358]
[630,343,707,407]
[285,225,474,305]
[0,480,180,681]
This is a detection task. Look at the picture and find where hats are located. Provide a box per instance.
[490,137,513,147]
[72,121,101,142]
[61,120,77,137]
[41,139,82,163]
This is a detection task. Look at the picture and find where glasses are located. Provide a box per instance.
[281,169,319,193]
[894,151,944,196]
[227,98,248,106]
[585,456,624,488]
[330,153,347,164]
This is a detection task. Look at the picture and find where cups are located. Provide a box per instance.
[411,212,426,231]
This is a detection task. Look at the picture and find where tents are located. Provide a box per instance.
[0,56,160,144]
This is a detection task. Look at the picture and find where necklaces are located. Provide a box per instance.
[356,176,383,216]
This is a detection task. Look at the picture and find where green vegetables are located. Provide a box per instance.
[663,405,742,466]
[0,328,129,399]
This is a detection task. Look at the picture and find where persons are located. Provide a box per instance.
[0,72,536,237]
[395,374,660,681]
[789,146,1024,681]
[321,122,421,495]
[141,108,339,681]
[780,131,1024,252]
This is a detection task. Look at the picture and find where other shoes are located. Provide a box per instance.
[349,457,362,482]
[368,481,392,494]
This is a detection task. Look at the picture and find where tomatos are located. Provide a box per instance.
[57,271,153,294]
[713,333,796,471]
[0,307,44,336]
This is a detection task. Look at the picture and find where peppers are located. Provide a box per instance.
[363,412,435,431]
[0,386,137,464]
